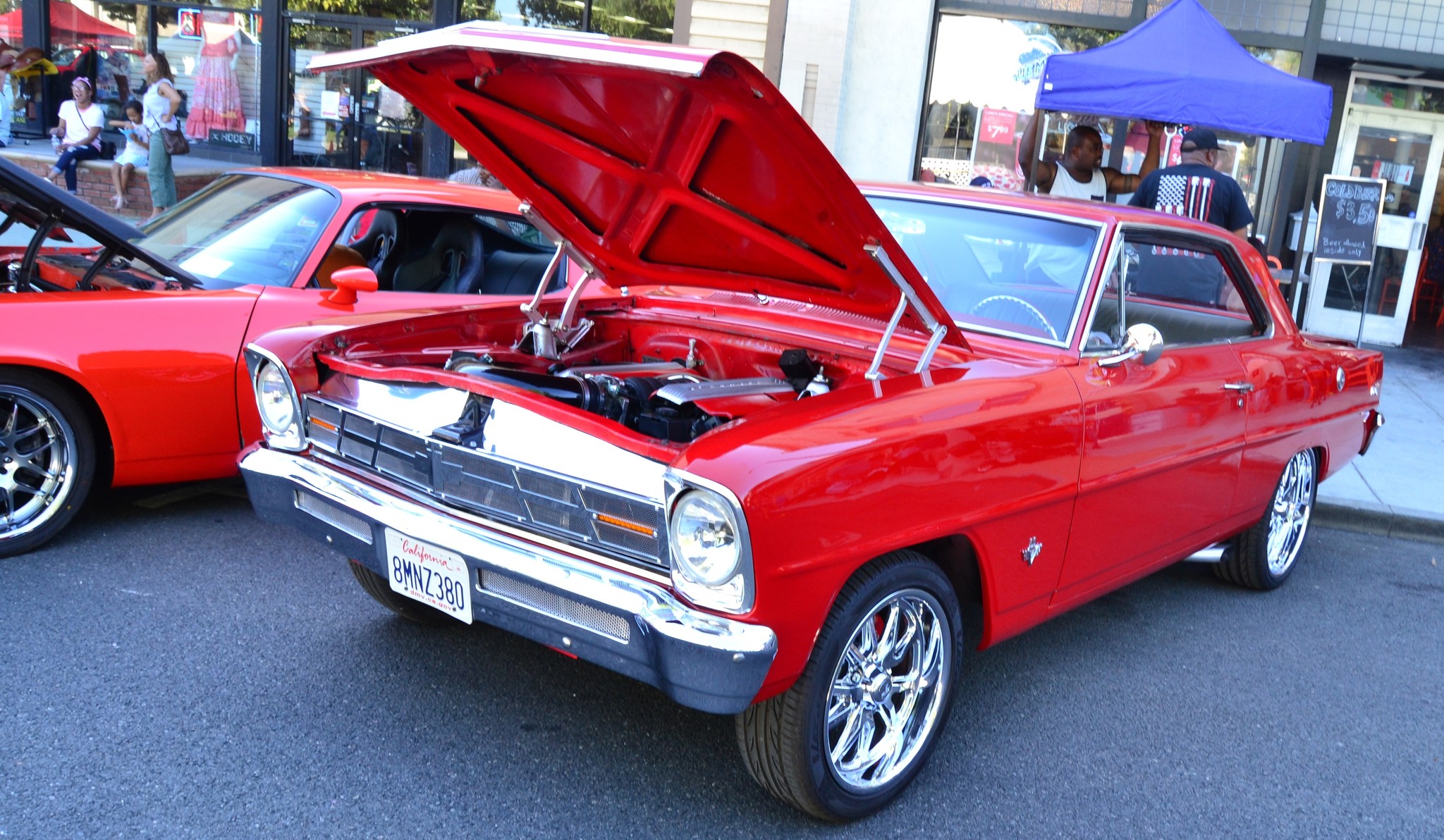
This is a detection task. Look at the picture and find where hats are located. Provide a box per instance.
[970,177,993,188]
[1180,127,1227,152]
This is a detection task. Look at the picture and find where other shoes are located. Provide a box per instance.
[132,79,148,95]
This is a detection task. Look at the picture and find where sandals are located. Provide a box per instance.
[110,195,117,203]
[114,196,128,209]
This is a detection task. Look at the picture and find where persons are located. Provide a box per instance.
[449,158,528,239]
[107,100,150,211]
[42,76,104,197]
[1115,127,1254,310]
[142,51,182,222]
[1017,107,1164,291]
[0,90,11,149]
[197,0,243,141]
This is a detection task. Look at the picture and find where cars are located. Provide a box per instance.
[233,19,1386,826]
[0,155,596,561]
[51,38,149,77]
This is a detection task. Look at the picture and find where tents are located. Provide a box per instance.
[1026,1,1333,316]
[0,0,145,58]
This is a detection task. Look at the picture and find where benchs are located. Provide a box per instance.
[480,247,560,295]
[949,279,1255,345]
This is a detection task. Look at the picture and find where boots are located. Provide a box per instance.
[297,108,310,137]
[174,90,190,119]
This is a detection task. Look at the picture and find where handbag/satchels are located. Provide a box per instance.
[160,122,190,155]
[99,140,117,160]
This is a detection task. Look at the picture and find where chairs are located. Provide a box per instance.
[1374,244,1434,320]
[1414,258,1439,313]
[391,216,486,295]
[342,207,401,291]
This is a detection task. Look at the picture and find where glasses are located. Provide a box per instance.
[71,86,89,92]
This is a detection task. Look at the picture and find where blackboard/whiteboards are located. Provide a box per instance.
[1313,173,1388,266]
